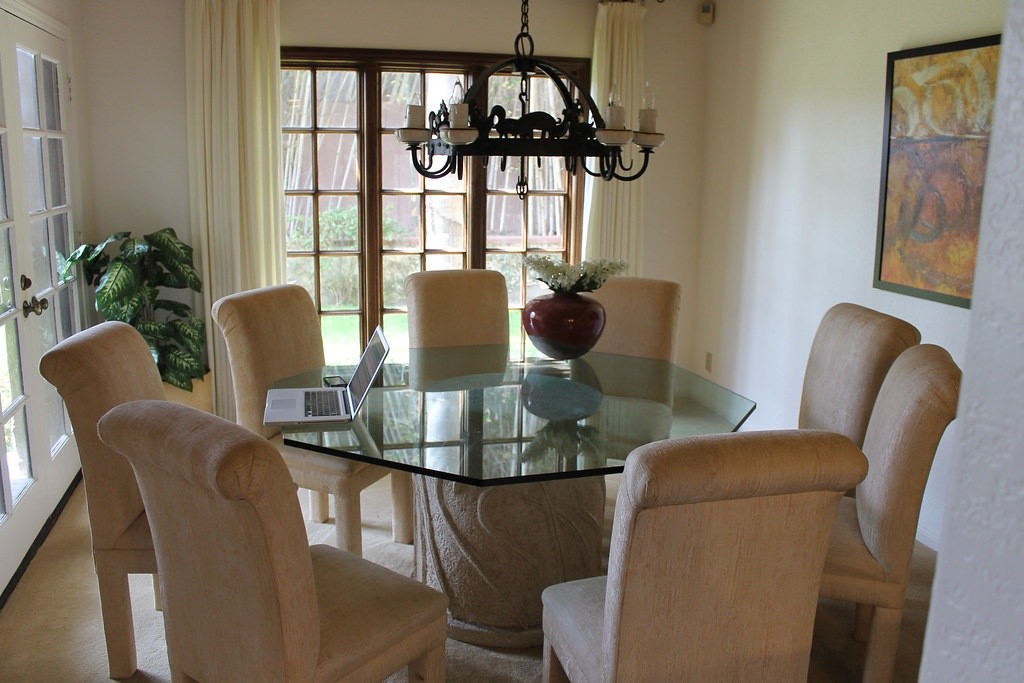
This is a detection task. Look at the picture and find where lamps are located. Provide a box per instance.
[394,0,664,200]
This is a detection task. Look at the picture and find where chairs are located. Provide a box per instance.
[210,284,415,561]
[819,345,963,683]
[404,268,510,348]
[796,303,921,497]
[40,321,299,679]
[98,399,447,683]
[580,278,683,366]
[541,429,870,683]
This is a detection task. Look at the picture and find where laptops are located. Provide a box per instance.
[263,323,389,426]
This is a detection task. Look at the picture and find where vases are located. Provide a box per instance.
[520,358,603,425]
[523,290,606,362]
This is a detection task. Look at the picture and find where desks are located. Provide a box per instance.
[273,353,759,650]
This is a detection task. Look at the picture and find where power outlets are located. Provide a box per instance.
[706,352,711,370]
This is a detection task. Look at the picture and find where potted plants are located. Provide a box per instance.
[59,227,214,418]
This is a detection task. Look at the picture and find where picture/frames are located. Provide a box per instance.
[872,33,1001,309]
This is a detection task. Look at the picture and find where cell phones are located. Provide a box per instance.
[323,376,347,387]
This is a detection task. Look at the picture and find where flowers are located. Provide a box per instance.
[518,421,607,463]
[521,254,627,294]
